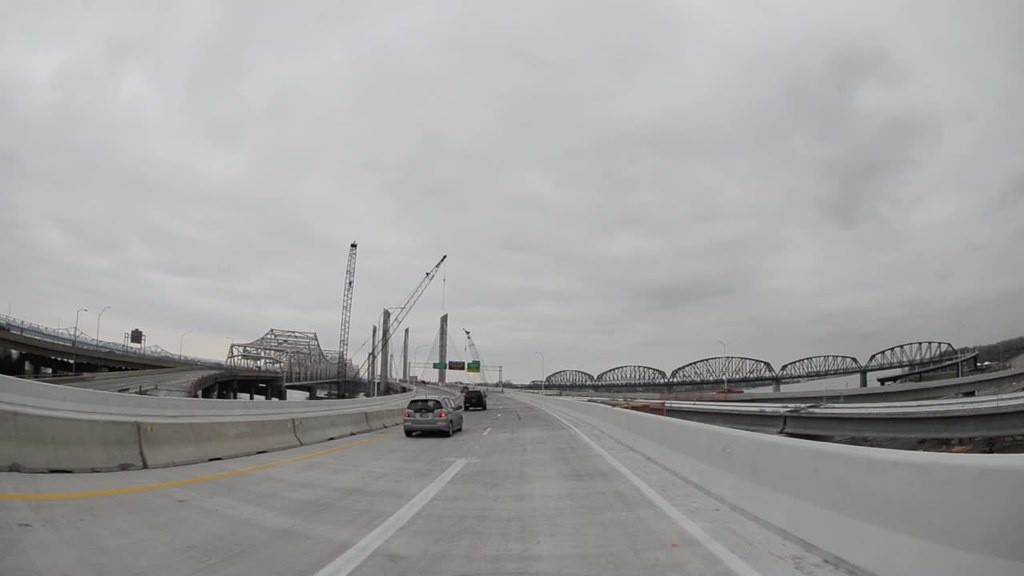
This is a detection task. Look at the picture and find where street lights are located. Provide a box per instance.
[72,308,88,374]
[95,305,111,351]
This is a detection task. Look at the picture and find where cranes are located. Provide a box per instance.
[323,239,448,400]
[462,328,491,387]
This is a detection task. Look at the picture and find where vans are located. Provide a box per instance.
[463,393,488,412]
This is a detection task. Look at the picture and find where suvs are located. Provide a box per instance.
[402,395,464,437]
